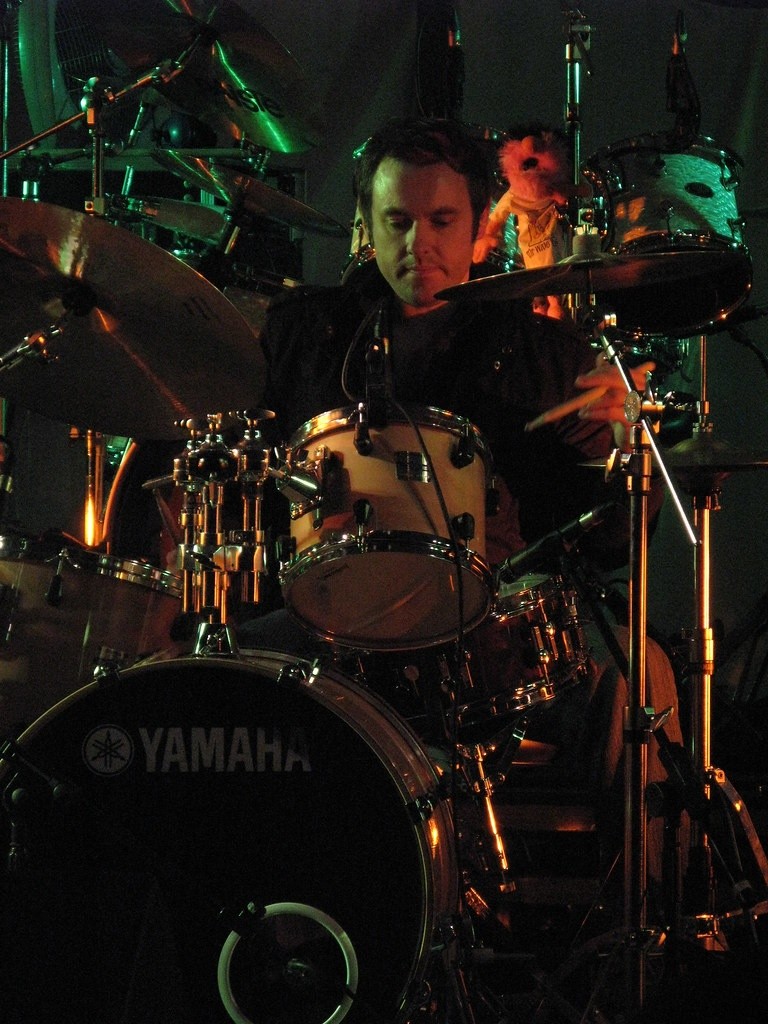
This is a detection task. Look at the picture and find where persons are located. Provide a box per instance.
[117,117,687,987]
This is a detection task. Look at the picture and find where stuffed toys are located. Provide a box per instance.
[472,136,571,321]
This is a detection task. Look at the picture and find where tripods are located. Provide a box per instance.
[496,322,768,1024]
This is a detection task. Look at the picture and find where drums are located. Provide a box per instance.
[570,131,757,335]
[283,396,499,654]
[0,646,462,1023]
[0,530,182,746]
[344,564,600,733]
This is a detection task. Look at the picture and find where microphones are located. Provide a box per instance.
[499,503,615,584]
[667,12,690,116]
[365,307,394,430]
[279,957,313,984]
[445,0,469,78]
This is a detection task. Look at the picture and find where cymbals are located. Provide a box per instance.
[433,248,727,302]
[0,195,270,441]
[149,150,352,241]
[83,4,327,157]
[578,431,768,492]
